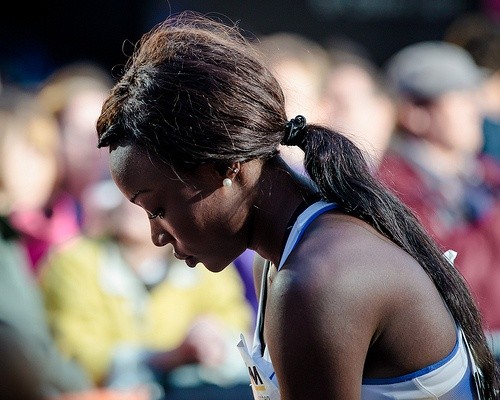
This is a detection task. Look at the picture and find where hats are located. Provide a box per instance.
[386,40,492,102]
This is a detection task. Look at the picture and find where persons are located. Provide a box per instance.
[0,70,264,399]
[252,29,498,367]
[95,12,499,400]
